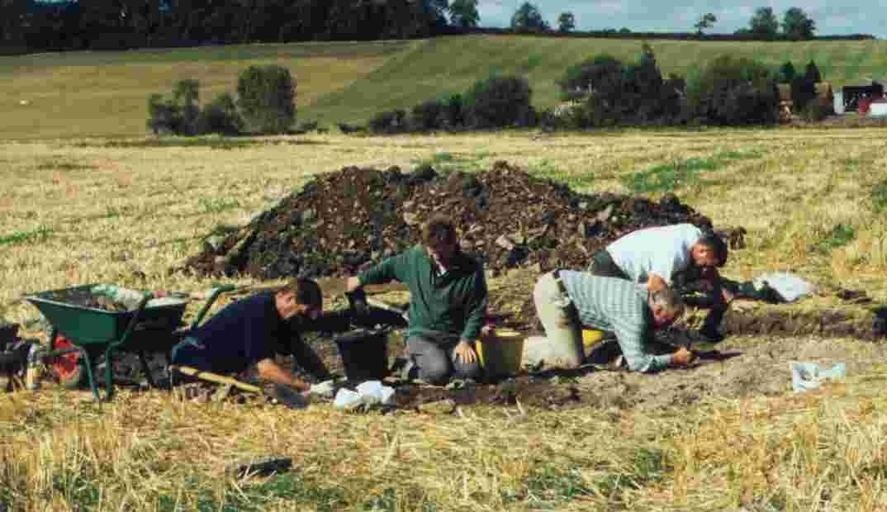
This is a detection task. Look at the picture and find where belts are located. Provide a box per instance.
[552,269,569,297]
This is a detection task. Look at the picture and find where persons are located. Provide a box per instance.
[517,268,692,372]
[587,223,735,342]
[25,328,101,393]
[346,219,487,385]
[165,278,334,398]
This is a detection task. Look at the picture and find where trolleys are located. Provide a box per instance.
[22,281,234,401]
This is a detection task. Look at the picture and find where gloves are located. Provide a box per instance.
[309,380,334,399]
